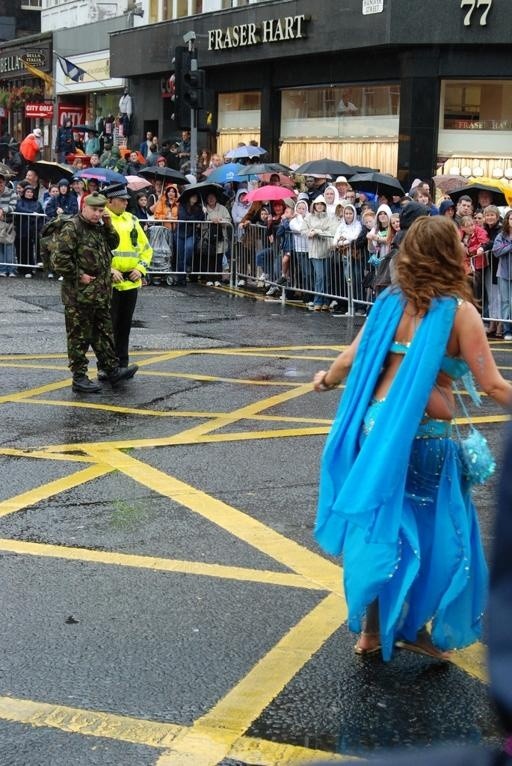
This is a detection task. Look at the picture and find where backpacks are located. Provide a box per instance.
[39,212,83,280]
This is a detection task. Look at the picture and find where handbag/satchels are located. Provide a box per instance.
[0,221,16,243]
[197,231,215,257]
[460,427,496,483]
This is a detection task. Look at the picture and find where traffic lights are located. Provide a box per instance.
[198,87,216,132]
[169,45,199,131]
[183,69,205,110]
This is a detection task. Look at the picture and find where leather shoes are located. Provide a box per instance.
[97,369,108,380]
[109,365,138,387]
[72,375,103,392]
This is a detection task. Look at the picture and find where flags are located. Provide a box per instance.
[57,54,87,83]
[23,62,54,93]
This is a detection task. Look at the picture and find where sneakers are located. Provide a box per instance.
[309,300,338,311]
[277,277,287,285]
[0,271,36,279]
[214,281,222,287]
[266,287,279,296]
[259,272,269,280]
[504,335,512,340]
[206,281,213,286]
[238,280,245,286]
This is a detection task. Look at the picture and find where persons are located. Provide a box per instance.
[314,215,512,663]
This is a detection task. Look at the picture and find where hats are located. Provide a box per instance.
[83,190,107,207]
[99,183,131,199]
[299,193,309,200]
[332,176,351,189]
[33,129,42,137]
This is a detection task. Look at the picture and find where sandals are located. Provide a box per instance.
[355,631,382,654]
[396,628,452,659]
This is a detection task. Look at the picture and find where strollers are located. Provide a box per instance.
[142,225,178,287]
